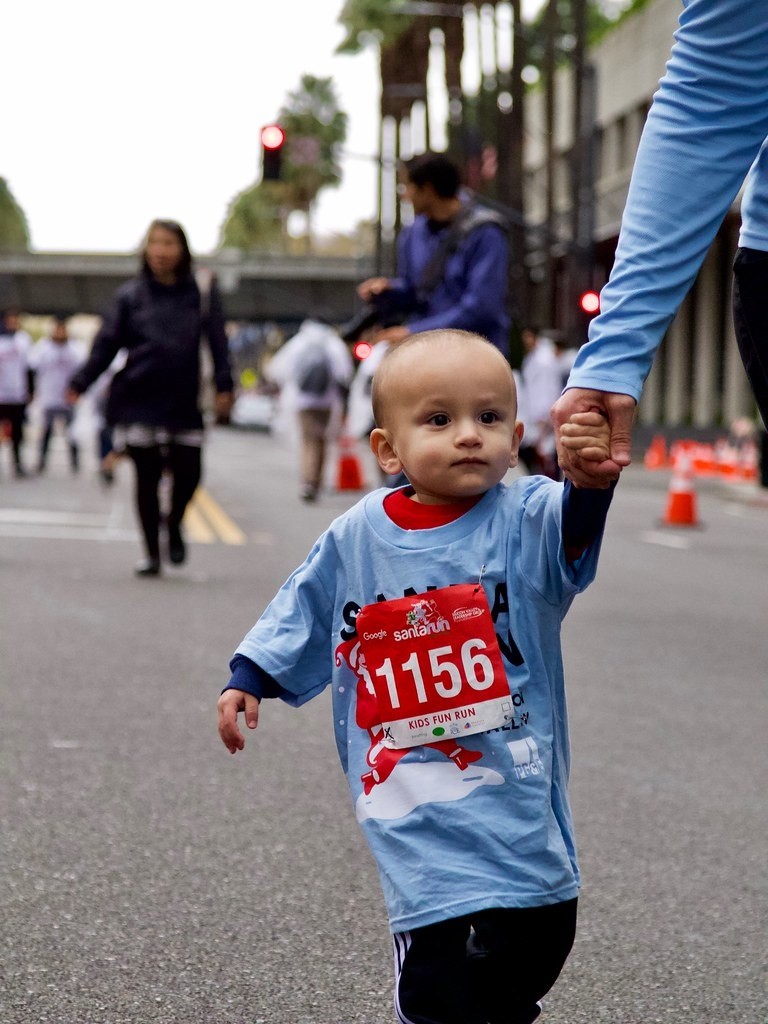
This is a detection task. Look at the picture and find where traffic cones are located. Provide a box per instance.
[644,432,758,489]
[337,439,368,491]
[660,450,700,526]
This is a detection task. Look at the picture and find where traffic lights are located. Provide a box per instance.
[577,283,600,322]
[260,126,284,178]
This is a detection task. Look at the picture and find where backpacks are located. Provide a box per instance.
[302,334,331,391]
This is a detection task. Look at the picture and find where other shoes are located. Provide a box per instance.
[165,516,185,564]
[135,557,160,577]
[299,481,320,501]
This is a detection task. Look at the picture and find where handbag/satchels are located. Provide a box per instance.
[340,286,435,353]
[341,341,391,444]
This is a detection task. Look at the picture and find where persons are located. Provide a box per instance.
[67,217,238,580]
[265,146,574,504]
[66,324,131,481]
[553,0,768,497]
[218,328,624,1024]
[25,307,91,473]
[0,303,35,476]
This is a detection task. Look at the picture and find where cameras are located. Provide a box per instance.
[340,277,413,344]
